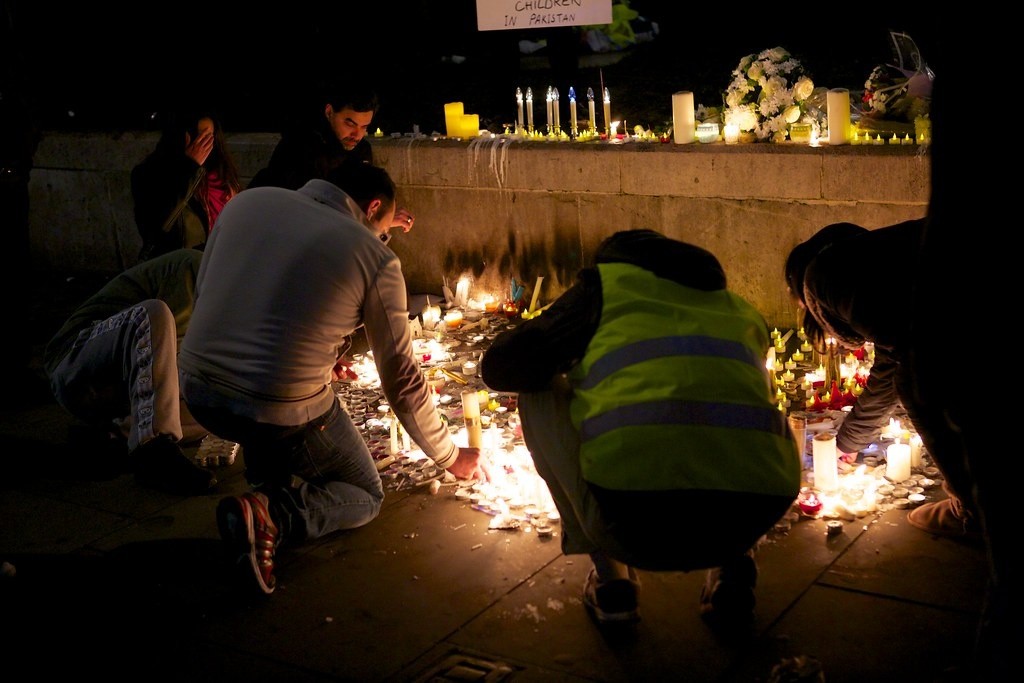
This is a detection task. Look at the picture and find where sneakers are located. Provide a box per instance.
[699,568,755,628]
[216,493,278,594]
[583,564,640,626]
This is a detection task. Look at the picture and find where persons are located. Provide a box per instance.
[132,99,241,260]
[480,229,801,626]
[180,164,479,594]
[786,218,1024,665]
[247,70,413,234]
[573,0,659,56]
[40,244,217,500]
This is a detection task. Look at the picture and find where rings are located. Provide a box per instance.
[408,216,411,222]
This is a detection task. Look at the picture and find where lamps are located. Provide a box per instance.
[696,122,720,143]
[788,122,812,143]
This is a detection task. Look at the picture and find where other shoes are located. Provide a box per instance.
[131,436,217,496]
[907,498,979,538]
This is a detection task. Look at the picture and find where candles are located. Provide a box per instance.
[917,134,928,145]
[444,102,464,136]
[851,133,861,145]
[827,88,850,145]
[873,134,884,145]
[672,92,695,145]
[725,125,738,144]
[889,133,900,144]
[501,85,670,144]
[335,273,935,536]
[902,133,913,145]
[195,433,239,467]
[862,132,873,145]
[462,114,479,139]
[375,128,383,137]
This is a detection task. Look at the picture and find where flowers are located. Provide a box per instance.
[698,46,820,143]
[862,64,933,115]
